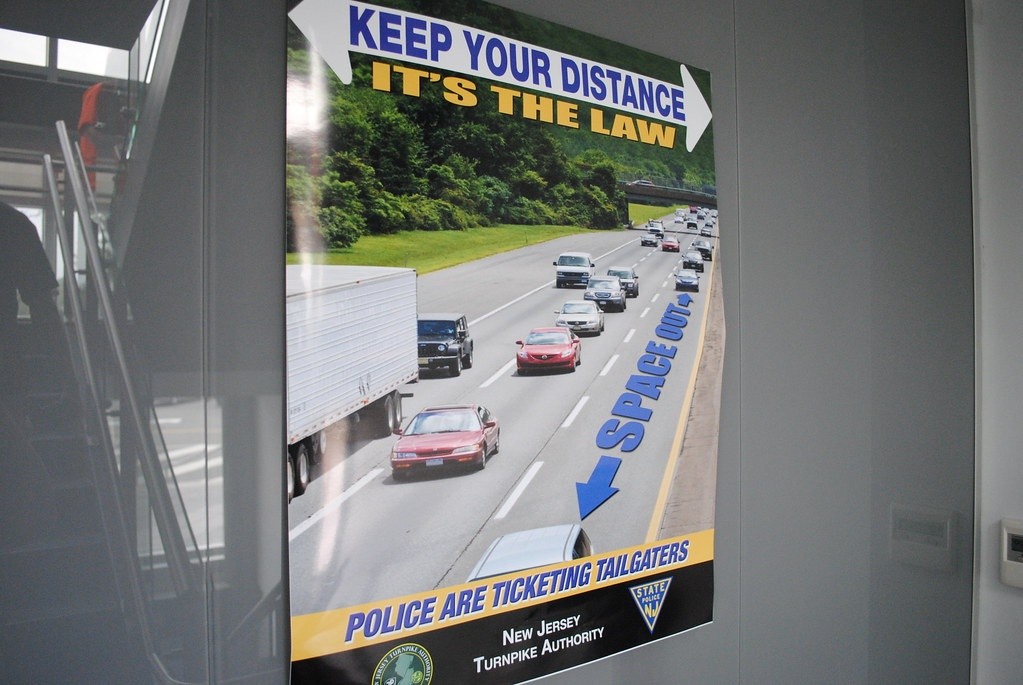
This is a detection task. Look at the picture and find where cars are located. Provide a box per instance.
[515,325,581,374]
[391,405,501,478]
[700,226,711,236]
[705,220,713,227]
[673,269,700,292]
[640,234,659,247]
[632,180,655,187]
[660,234,680,252]
[674,217,684,224]
[689,206,718,224]
[553,301,605,335]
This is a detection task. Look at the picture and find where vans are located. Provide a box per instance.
[553,253,595,288]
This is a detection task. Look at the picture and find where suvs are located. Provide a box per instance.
[682,250,704,273]
[686,217,698,230]
[417,312,474,378]
[584,275,627,313]
[645,219,665,239]
[608,267,640,298]
[692,240,714,261]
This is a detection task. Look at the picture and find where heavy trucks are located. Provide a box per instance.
[285,261,419,501]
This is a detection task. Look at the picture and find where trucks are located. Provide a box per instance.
[675,209,688,221]
